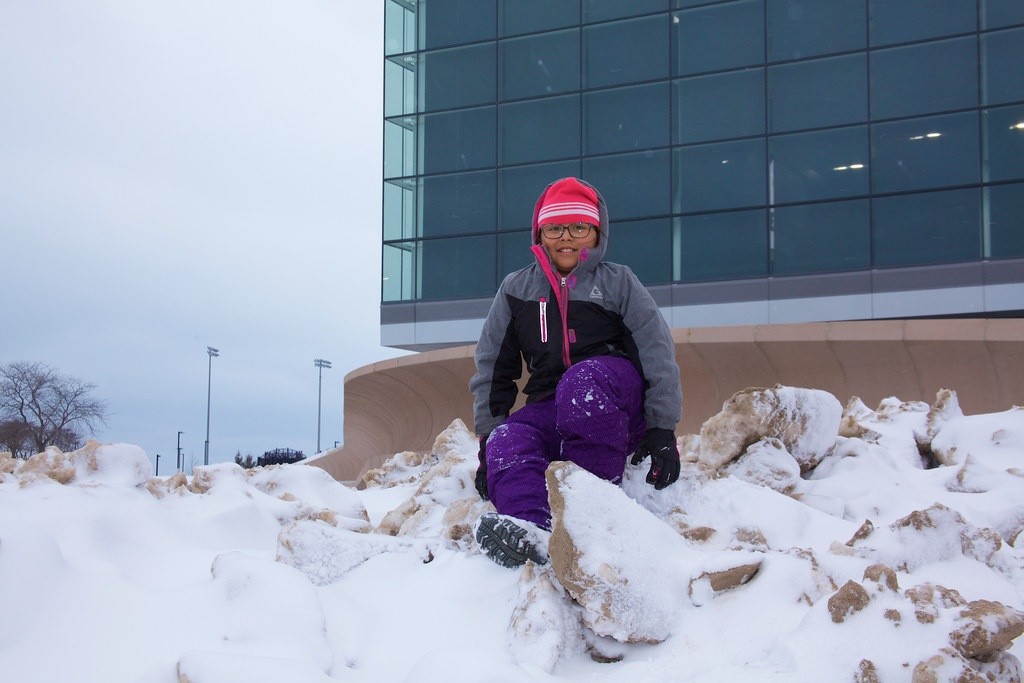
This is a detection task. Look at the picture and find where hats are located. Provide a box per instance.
[537,177,600,230]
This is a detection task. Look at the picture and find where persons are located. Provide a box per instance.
[467,177,681,571]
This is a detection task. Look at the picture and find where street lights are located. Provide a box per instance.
[156,454,162,476]
[205,441,208,465]
[206,346,219,465]
[177,431,184,472]
[180,454,184,472]
[314,359,332,453]
[178,448,182,472]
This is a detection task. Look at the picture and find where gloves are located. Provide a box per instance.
[473,434,490,501]
[632,427,681,490]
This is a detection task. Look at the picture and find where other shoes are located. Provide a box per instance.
[471,511,550,569]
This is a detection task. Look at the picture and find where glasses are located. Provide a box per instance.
[541,222,592,239]
